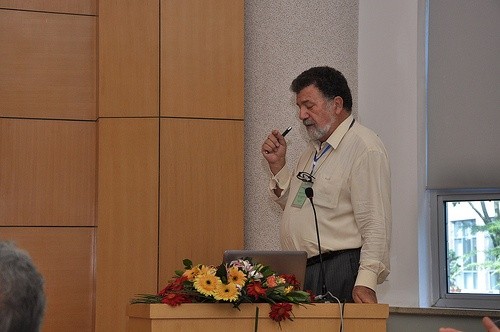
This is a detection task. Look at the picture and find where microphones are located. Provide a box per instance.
[305,186,336,303]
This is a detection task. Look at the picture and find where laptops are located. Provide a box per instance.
[222,250,307,291]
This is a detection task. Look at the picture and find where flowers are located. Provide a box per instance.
[130,256,317,332]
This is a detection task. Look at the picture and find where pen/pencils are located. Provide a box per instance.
[266,126,292,154]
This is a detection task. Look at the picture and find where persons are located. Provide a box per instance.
[439,316,500,332]
[262,66,391,304]
[0,241,47,332]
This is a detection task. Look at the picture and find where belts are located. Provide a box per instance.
[307,250,341,266]
